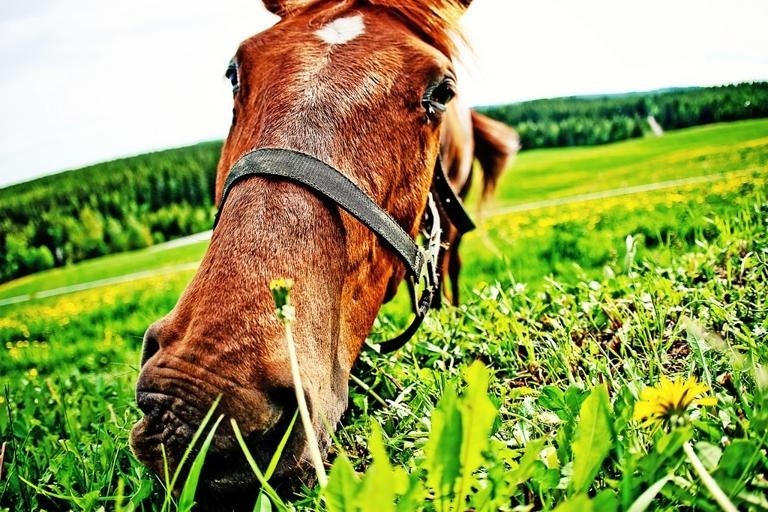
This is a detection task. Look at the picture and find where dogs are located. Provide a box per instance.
[128,0,522,512]
